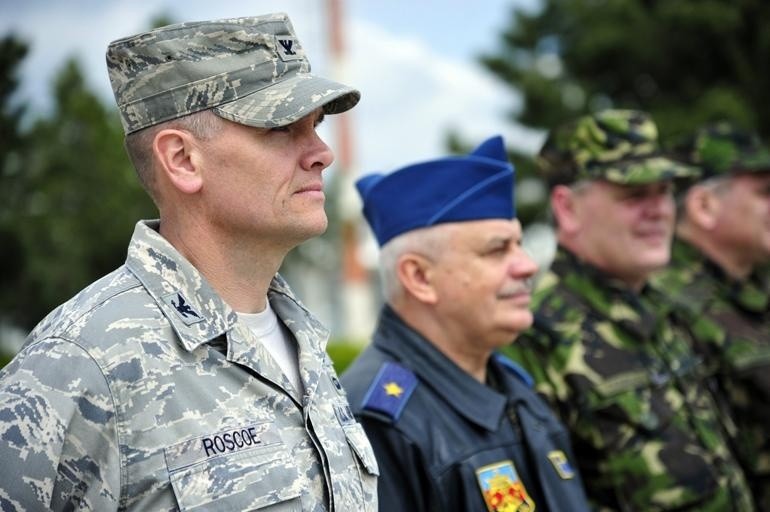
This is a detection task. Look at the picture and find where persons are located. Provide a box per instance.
[336,135,591,511]
[493,107,769,512]
[647,84,769,419]
[0,12,380,512]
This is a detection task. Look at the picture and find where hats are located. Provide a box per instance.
[543,108,768,184]
[106,13,360,137]
[355,136,516,247]
